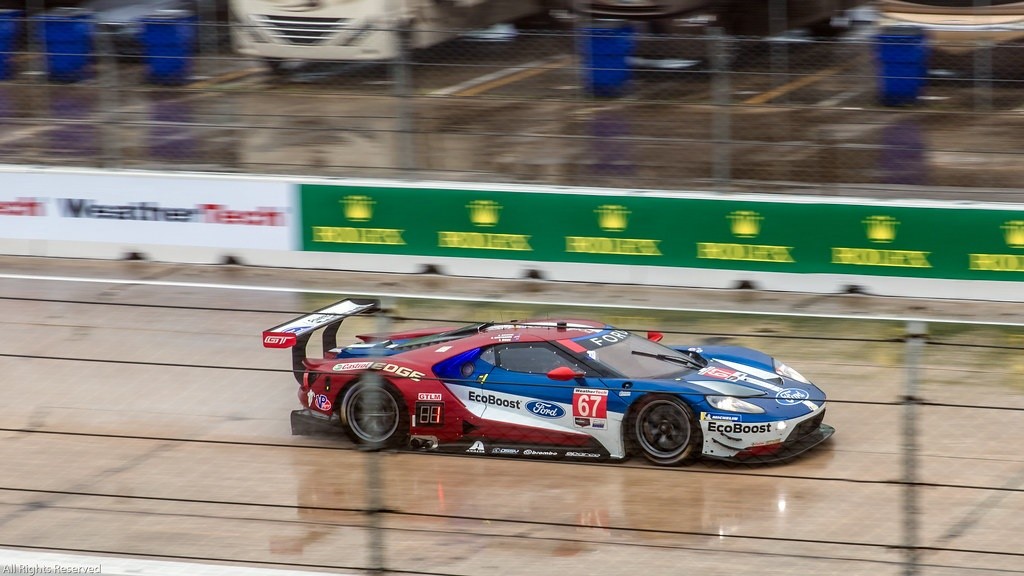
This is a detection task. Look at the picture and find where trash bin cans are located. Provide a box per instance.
[0,8,23,82]
[869,24,935,104]
[575,17,636,96]
[133,8,199,85]
[34,7,97,83]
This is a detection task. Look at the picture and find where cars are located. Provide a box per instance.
[226,1,553,69]
[567,0,861,63]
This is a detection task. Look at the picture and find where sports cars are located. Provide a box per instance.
[258,295,837,471]
